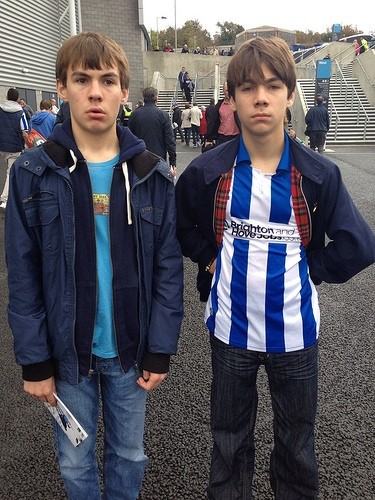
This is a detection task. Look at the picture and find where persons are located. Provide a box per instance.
[0,89,26,208]
[178,67,187,96]
[180,103,193,146]
[120,104,132,125]
[127,86,178,177]
[203,81,241,152]
[28,99,58,141]
[183,76,193,104]
[151,29,372,55]
[177,36,375,500]
[5,31,185,499]
[171,102,186,144]
[304,96,331,155]
[188,102,202,147]
[286,128,305,146]
[16,99,34,125]
[50,98,60,114]
[205,98,217,126]
[198,106,208,145]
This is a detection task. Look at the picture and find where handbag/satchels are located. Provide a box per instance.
[22,116,48,149]
[304,124,313,137]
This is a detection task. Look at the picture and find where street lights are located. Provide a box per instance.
[157,17,167,47]
